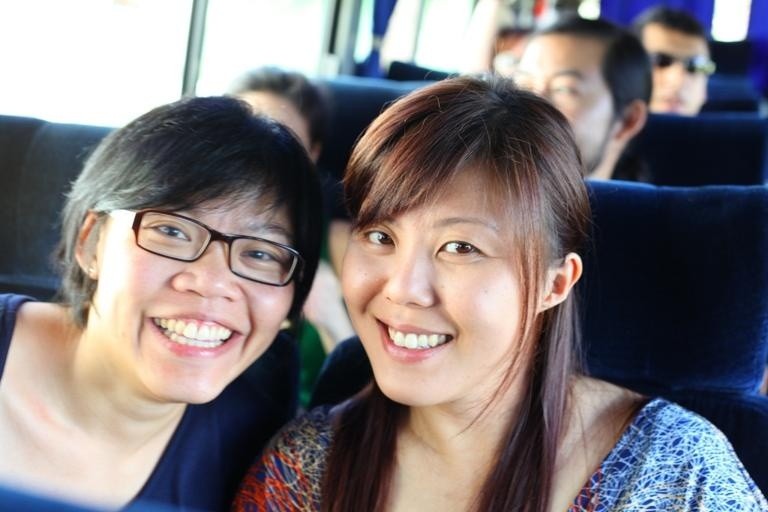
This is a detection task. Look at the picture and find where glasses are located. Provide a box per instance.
[103,209,309,287]
[649,52,717,75]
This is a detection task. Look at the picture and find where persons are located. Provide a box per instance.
[231,64,356,417]
[462,0,537,79]
[228,75,768,512]
[0,97,326,512]
[628,6,717,118]
[512,17,653,181]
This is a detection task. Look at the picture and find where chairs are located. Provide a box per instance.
[0,40,768,512]
